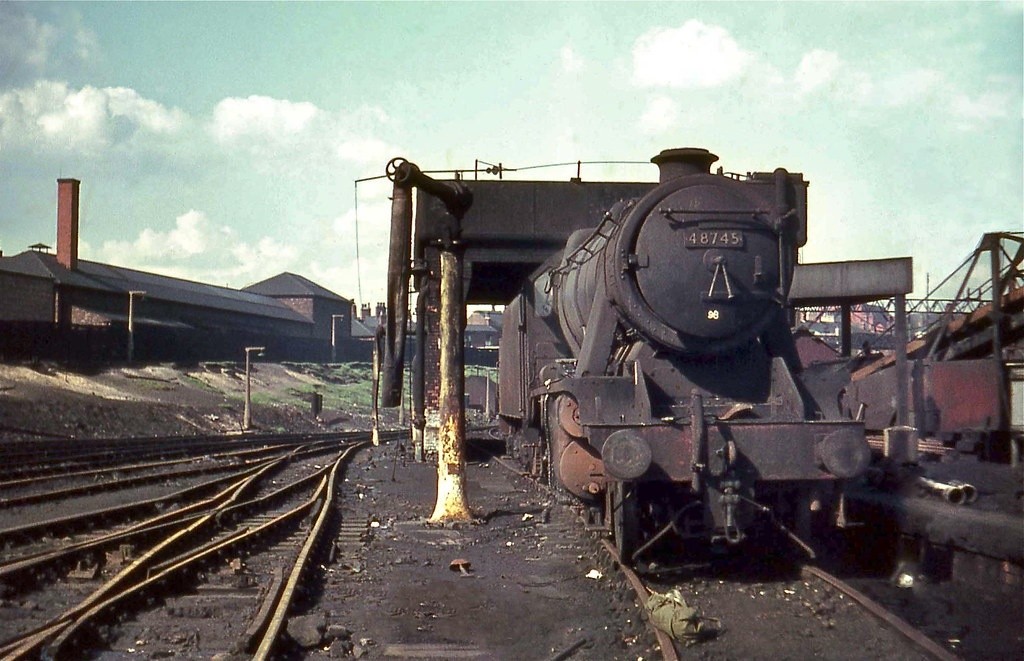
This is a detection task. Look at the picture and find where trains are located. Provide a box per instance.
[500,148,872,565]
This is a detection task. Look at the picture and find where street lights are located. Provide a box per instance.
[331,315,344,363]
[128,291,147,366]
[245,347,266,429]
